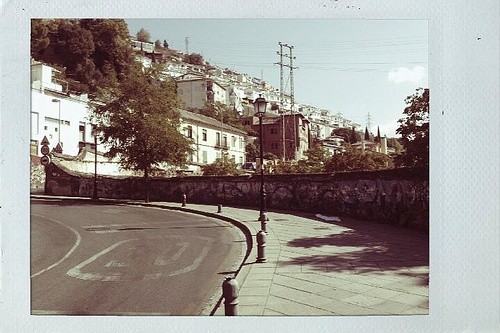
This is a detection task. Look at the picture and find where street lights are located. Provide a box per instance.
[91,125,101,199]
[253,97,269,222]
[52,99,62,151]
